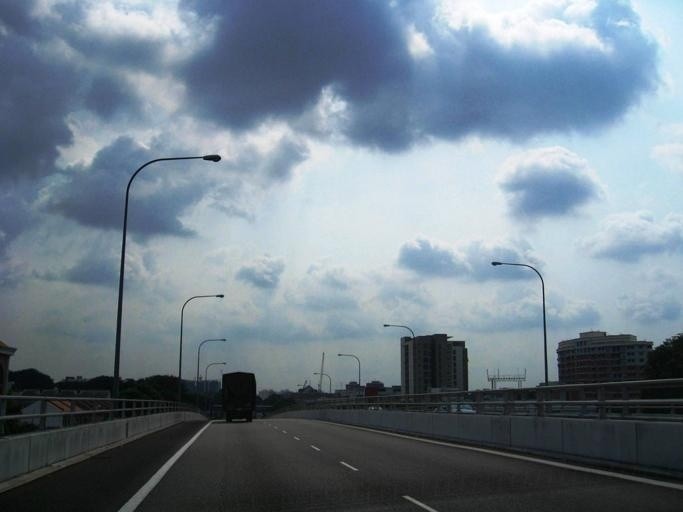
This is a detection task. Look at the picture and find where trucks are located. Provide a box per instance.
[222,372,256,422]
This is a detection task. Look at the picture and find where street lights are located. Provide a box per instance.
[177,294,224,401]
[112,154,220,399]
[313,373,331,394]
[196,339,226,408]
[338,354,360,396]
[205,363,227,406]
[384,324,416,395]
[492,263,547,386]
